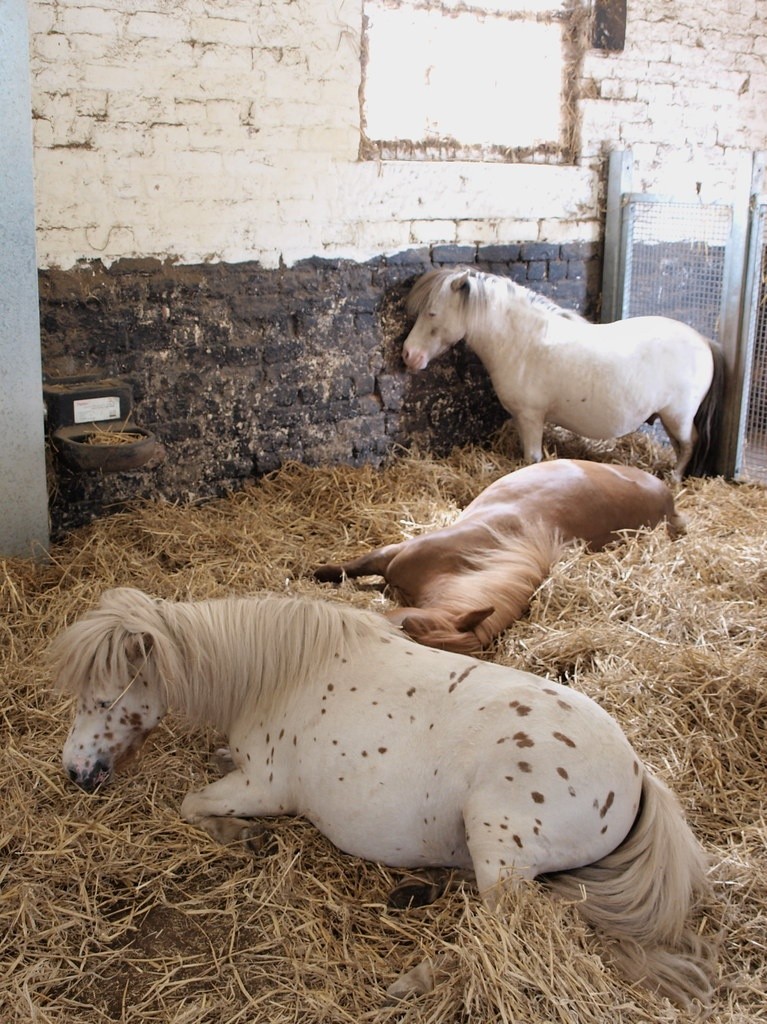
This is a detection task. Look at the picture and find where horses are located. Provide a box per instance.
[402,259,728,481]
[36,585,727,1007]
[308,458,686,654]
